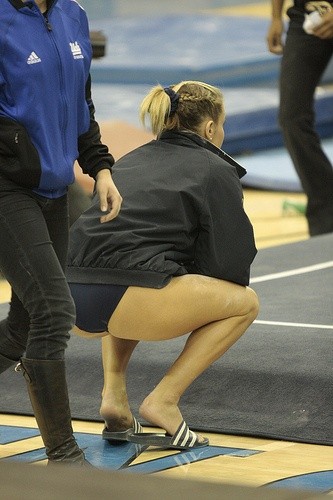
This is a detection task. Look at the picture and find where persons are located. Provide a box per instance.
[67,81,260,449]
[0,0,123,469]
[267,0,333,237]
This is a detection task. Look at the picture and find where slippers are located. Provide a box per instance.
[127,419,209,449]
[103,417,143,441]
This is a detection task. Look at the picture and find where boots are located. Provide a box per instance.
[0,354,18,374]
[15,357,99,470]
